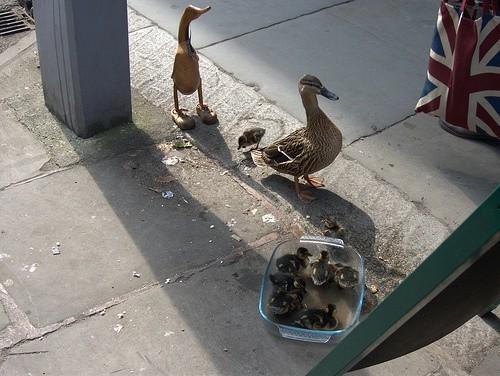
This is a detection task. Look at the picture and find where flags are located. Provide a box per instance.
[414,3,499,140]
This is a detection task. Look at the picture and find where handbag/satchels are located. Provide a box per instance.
[411,0,500,141]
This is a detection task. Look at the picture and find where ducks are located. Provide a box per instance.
[321,216,348,258]
[237,126,266,151]
[266,246,359,331]
[258,73,343,204]
[171,4,218,130]
